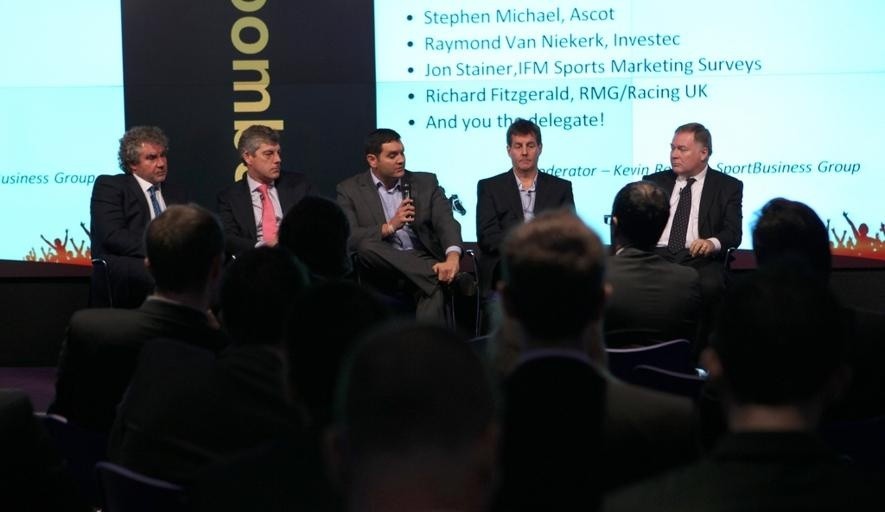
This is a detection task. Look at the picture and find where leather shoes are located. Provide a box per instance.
[447,269,480,295]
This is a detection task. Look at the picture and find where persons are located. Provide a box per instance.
[1,180,885,510]
[217,124,312,259]
[477,120,578,319]
[613,121,743,298]
[90,127,191,302]
[336,128,476,330]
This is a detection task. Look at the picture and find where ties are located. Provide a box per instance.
[147,185,163,220]
[668,177,698,258]
[257,184,279,245]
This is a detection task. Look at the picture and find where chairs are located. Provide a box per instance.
[85,459,187,511]
[25,409,96,510]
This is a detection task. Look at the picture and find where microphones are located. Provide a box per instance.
[401,179,416,219]
